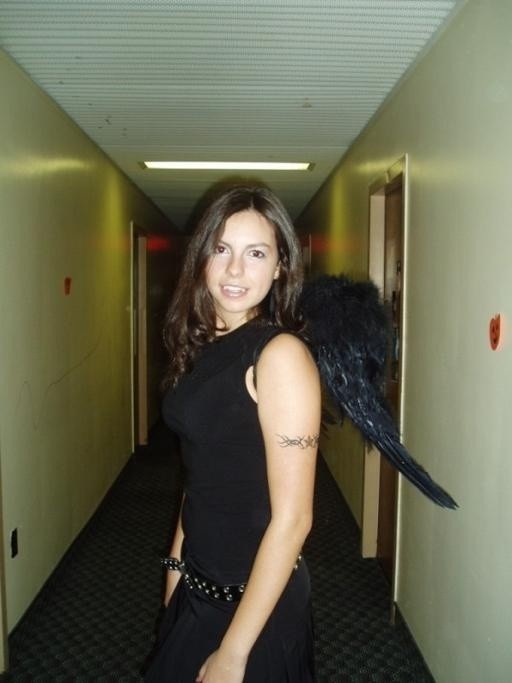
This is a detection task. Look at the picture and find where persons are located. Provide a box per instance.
[143,173,329,681]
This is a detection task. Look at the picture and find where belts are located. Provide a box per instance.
[162,553,306,608]
